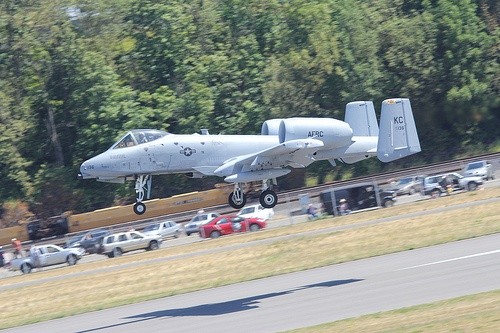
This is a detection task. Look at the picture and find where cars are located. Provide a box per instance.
[199,214,267,239]
[237,202,274,220]
[182,213,220,237]
[141,220,182,241]
[68,228,111,254]
[102,232,163,257]
[423,171,483,199]
[384,174,428,196]
[462,160,496,181]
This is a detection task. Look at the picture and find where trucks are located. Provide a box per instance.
[320,182,398,215]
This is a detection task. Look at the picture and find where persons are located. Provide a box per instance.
[11,238,23,257]
[307,204,319,219]
[138,132,147,143]
[438,174,450,189]
[339,198,348,215]
[31,248,41,271]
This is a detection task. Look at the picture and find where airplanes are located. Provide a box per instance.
[77,98,423,215]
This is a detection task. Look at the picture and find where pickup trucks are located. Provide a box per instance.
[10,244,85,274]
